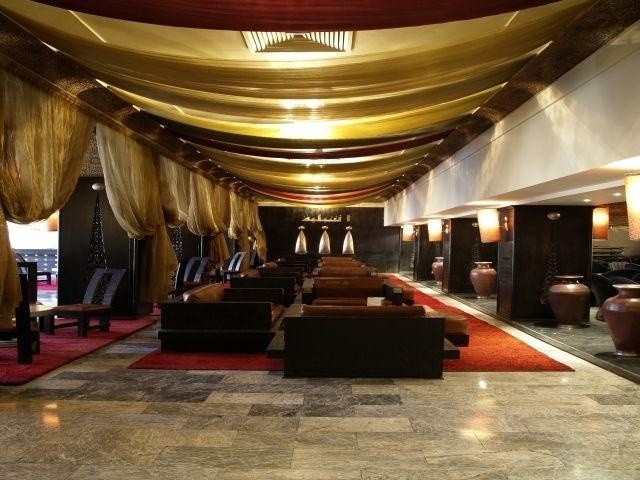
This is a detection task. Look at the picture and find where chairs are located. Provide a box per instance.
[57,267,128,336]
[183,256,209,288]
[228,252,246,273]
[0,275,39,357]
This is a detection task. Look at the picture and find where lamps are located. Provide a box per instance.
[591,204,614,243]
[426,216,451,244]
[477,208,510,245]
[400,224,419,243]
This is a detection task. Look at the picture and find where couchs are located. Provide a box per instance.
[303,278,401,307]
[158,282,284,352]
[314,257,370,278]
[283,305,446,379]
[241,260,307,292]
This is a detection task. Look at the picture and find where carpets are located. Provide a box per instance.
[1,279,160,385]
[129,271,574,374]
